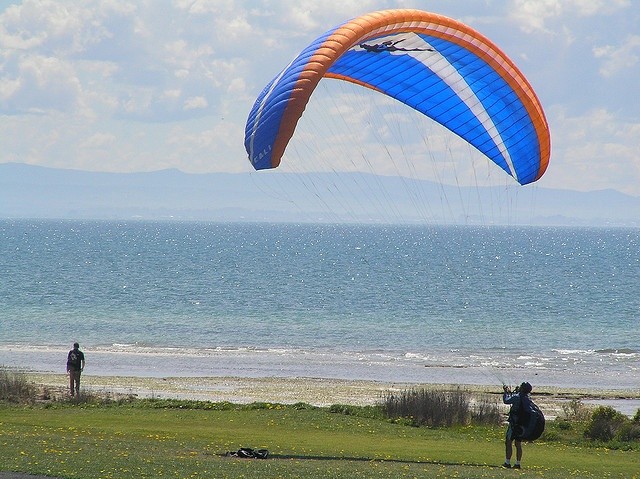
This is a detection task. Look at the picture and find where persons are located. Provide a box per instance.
[503,381,532,468]
[65,342,85,397]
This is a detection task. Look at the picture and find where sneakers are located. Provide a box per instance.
[502,462,511,468]
[511,464,520,468]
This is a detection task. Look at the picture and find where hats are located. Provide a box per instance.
[522,382,532,393]
